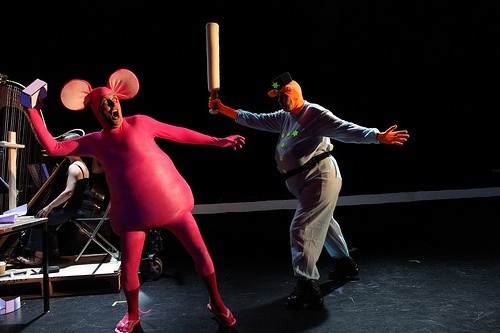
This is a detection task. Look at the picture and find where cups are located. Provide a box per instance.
[0,261,6,275]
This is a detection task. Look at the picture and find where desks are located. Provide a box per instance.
[0,215,50,312]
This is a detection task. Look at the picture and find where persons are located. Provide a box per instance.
[21,67,246,333]
[17,132,92,267]
[207,72,410,308]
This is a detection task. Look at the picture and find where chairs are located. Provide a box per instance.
[65,196,124,263]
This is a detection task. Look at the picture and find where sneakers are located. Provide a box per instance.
[279,278,324,307]
[327,256,359,281]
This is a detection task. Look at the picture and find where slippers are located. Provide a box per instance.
[206,302,237,327]
[114,314,141,333]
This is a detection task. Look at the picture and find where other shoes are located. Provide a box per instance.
[50,247,60,261]
[19,252,44,267]
